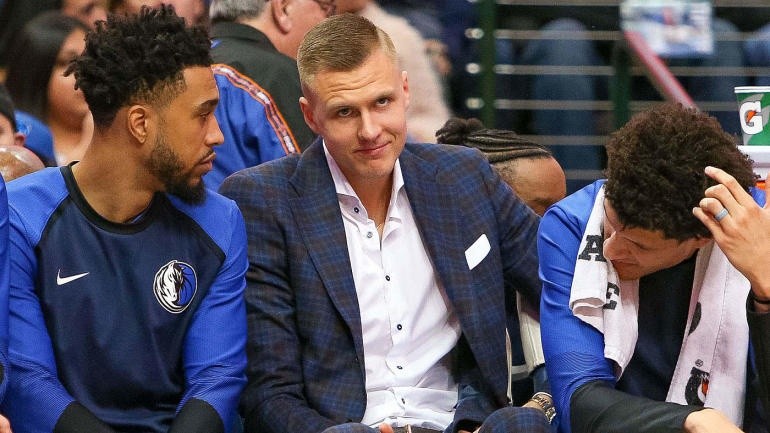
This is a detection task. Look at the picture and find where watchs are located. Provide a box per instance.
[529,393,557,425]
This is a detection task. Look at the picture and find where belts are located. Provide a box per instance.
[393,424,444,433]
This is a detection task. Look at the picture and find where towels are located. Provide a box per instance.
[567,179,752,431]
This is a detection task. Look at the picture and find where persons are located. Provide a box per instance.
[0,0,453,192]
[0,4,250,433]
[538,102,770,433]
[435,117,567,218]
[218,13,557,433]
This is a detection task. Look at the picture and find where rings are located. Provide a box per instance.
[715,208,728,224]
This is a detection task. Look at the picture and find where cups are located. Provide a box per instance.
[734,87,770,145]
